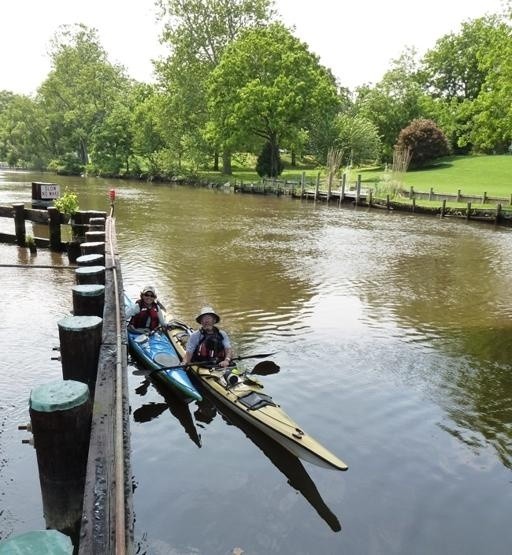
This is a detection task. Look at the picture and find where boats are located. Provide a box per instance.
[127,302,349,472]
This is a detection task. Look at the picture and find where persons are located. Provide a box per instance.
[125,285,167,334]
[180,308,233,369]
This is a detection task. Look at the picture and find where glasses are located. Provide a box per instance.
[145,294,155,297]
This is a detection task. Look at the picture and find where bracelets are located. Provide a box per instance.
[225,357,230,361]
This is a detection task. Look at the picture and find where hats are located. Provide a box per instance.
[141,286,155,295]
[196,307,219,323]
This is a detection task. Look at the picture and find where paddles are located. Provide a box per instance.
[133,350,280,375]
[134,315,172,343]
